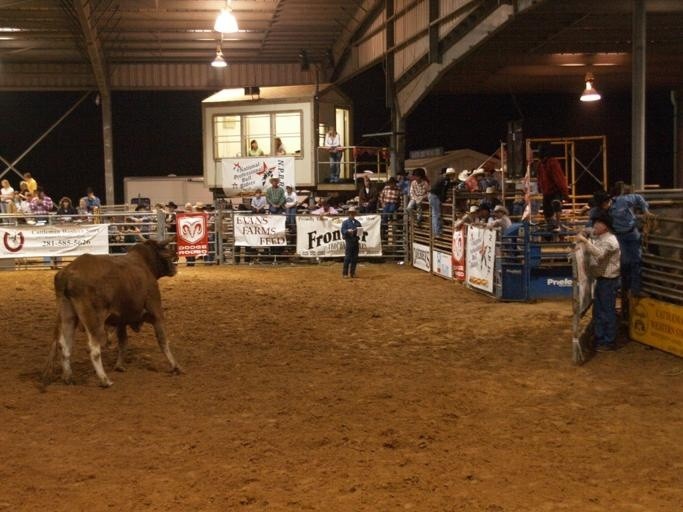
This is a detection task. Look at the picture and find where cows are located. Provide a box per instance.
[41,231,185,385]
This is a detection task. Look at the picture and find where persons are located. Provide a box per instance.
[324,127,342,184]
[110,201,226,266]
[234,175,347,265]
[575,179,651,351]
[341,207,362,278]
[0,172,102,270]
[360,166,534,248]
[248,139,263,157]
[537,142,570,232]
[276,138,285,155]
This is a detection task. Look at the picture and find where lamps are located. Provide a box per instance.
[578,69,601,101]
[209,40,227,68]
[213,0,238,34]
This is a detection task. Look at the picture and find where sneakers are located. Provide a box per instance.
[595,345,617,353]
[343,274,354,279]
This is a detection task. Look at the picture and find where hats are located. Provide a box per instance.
[472,169,488,176]
[348,207,358,214]
[387,178,400,183]
[270,176,280,184]
[442,167,457,175]
[458,170,470,181]
[494,204,509,215]
[592,212,617,232]
[477,204,491,213]
